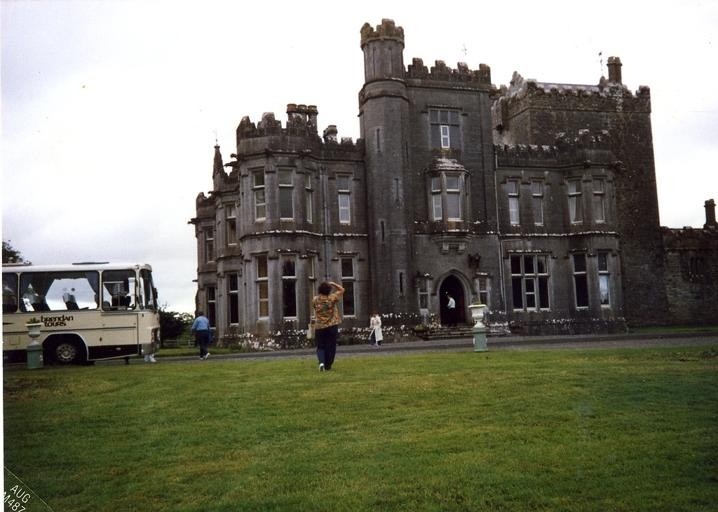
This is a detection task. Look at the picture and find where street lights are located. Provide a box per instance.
[306,322,315,340]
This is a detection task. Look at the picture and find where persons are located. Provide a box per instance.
[191,311,214,360]
[367,311,384,347]
[447,294,457,326]
[309,280,346,371]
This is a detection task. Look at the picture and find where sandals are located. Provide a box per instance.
[2,261,162,366]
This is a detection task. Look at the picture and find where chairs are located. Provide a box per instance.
[318,362,325,373]
[144,353,211,364]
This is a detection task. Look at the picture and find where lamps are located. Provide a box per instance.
[3,289,110,313]
